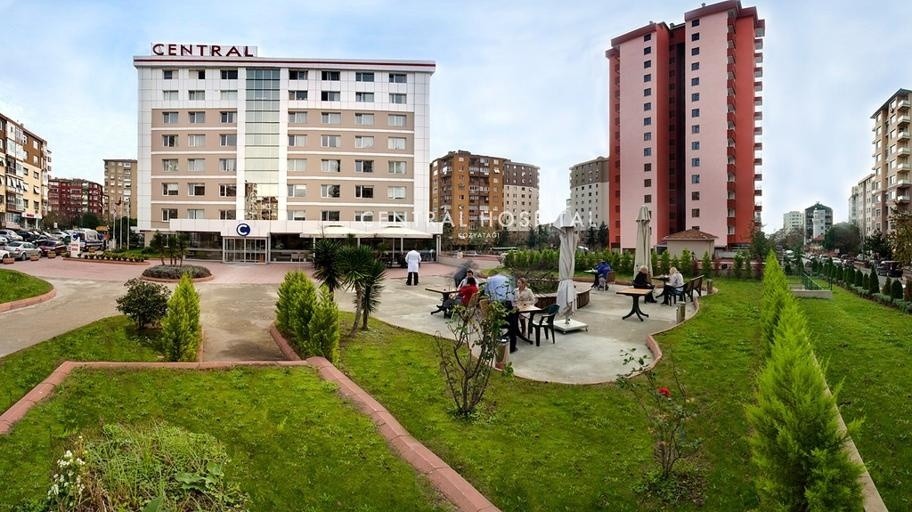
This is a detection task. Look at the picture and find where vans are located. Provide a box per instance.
[875,260,904,278]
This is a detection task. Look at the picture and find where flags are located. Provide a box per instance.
[111,207,117,217]
[115,197,121,205]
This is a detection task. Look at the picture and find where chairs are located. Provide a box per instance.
[630,279,655,304]
[479,298,559,346]
[669,275,704,304]
[308,254,314,262]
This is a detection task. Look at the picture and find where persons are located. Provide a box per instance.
[634,265,657,302]
[436,264,538,336]
[661,267,683,305]
[405,247,423,285]
[591,259,611,290]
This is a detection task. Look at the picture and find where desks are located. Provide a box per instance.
[426,286,460,318]
[584,270,616,290]
[617,288,652,321]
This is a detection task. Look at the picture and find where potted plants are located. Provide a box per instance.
[30,250,39,261]
[88,246,96,253]
[48,251,56,258]
[61,249,68,257]
[304,254,307,262]
[3,254,14,264]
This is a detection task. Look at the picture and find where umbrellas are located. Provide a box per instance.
[633,206,653,280]
[553,210,577,324]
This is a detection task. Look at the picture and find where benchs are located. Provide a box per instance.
[290,253,304,262]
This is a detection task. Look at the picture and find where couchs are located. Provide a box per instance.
[276,252,298,261]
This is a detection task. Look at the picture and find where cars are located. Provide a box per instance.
[0,226,107,264]
[784,248,878,268]
